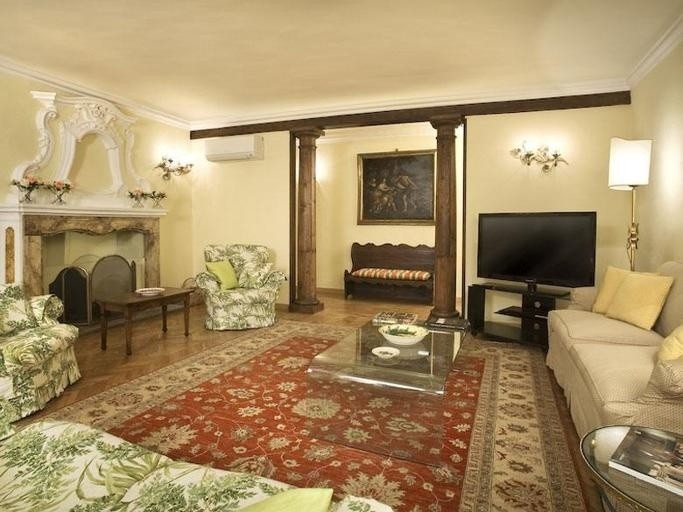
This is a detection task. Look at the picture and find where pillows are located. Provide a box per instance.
[603,273,674,331]
[658,325,682,362]
[204,259,238,290]
[239,261,274,289]
[0,281,38,336]
[591,266,628,314]
[238,488,334,512]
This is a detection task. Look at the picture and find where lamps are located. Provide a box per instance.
[609,136,652,271]
[511,140,569,173]
[155,155,194,181]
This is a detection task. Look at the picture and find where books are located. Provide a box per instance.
[372,310,418,325]
[608,426,683,497]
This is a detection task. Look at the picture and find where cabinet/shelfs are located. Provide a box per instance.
[468,282,571,349]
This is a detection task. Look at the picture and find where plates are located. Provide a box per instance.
[378,324,429,346]
[136,287,165,297]
[372,346,400,359]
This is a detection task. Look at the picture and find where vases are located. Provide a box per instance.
[153,202,163,208]
[132,201,144,208]
[50,197,67,206]
[18,196,37,204]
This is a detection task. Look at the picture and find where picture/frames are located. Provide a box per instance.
[356,149,436,226]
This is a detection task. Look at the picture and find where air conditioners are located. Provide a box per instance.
[204,135,264,162]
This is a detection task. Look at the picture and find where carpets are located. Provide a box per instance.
[27,318,612,511]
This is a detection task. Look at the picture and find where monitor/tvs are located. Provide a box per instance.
[477,211,597,297]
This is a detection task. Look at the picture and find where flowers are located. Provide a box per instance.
[43,178,72,199]
[147,191,167,202]
[10,175,44,196]
[129,189,148,200]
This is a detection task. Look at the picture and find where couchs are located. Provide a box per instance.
[196,244,287,331]
[0,397,362,512]
[344,242,436,306]
[0,294,82,423]
[547,260,683,512]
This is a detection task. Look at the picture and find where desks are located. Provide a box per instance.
[95,287,195,356]
